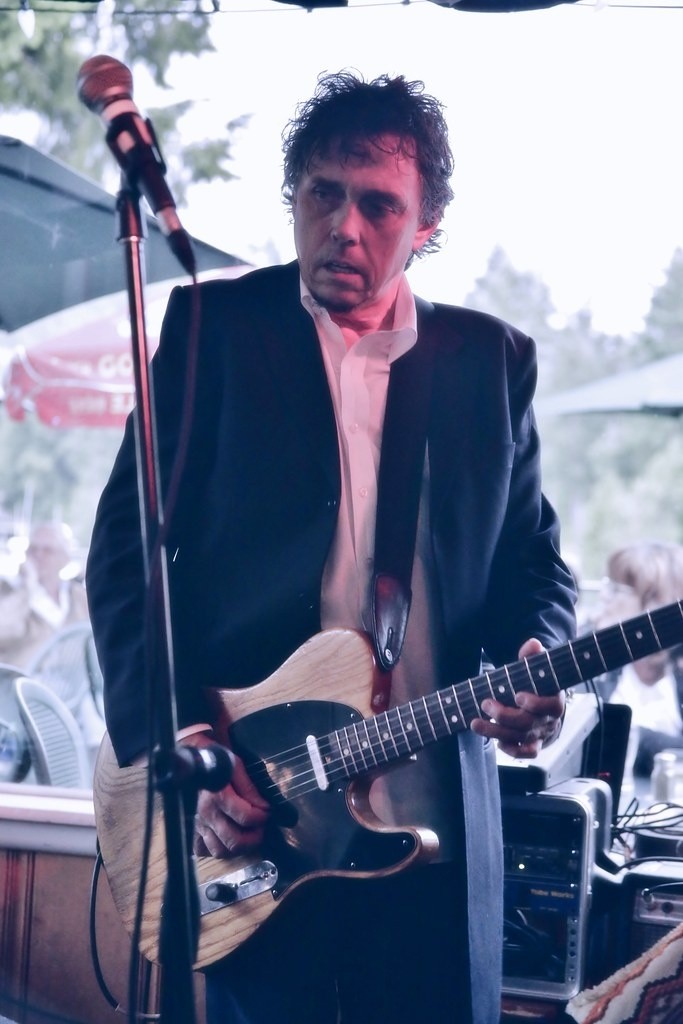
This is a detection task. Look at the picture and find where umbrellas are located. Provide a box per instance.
[0,132,251,334]
[545,344,683,419]
[0,265,259,432]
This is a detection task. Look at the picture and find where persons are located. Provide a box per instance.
[572,541,683,853]
[84,65,578,1024]
[1,524,88,675]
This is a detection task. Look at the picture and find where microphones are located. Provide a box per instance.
[76,54,198,277]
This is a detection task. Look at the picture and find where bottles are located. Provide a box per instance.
[650,753,676,801]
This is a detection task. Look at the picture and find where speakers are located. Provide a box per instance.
[609,861,683,980]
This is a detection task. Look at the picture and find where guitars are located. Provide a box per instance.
[91,598,682,973]
